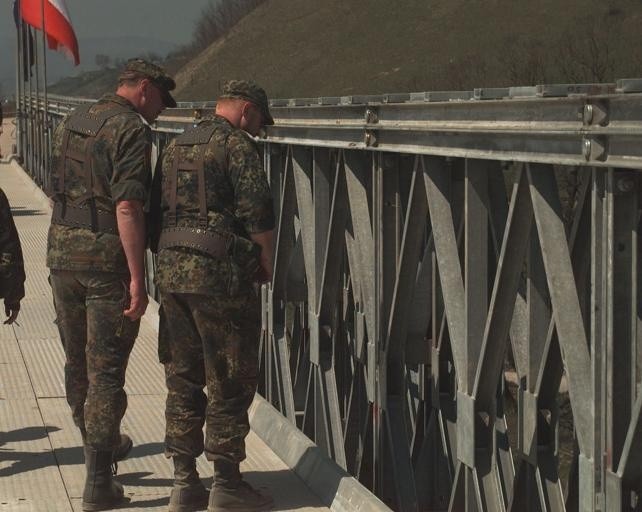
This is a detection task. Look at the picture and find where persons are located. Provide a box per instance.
[0,189,26,324]
[47,58,177,511]
[150,79,275,511]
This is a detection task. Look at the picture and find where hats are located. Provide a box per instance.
[218,79,274,125]
[126,59,177,108]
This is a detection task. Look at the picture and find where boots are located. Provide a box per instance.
[78,426,132,461]
[167,456,211,511]
[82,445,123,511]
[208,461,274,511]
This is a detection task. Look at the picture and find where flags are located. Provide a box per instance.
[20,0,80,65]
[14,0,35,81]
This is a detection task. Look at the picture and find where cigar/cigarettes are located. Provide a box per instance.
[9,317,20,326]
